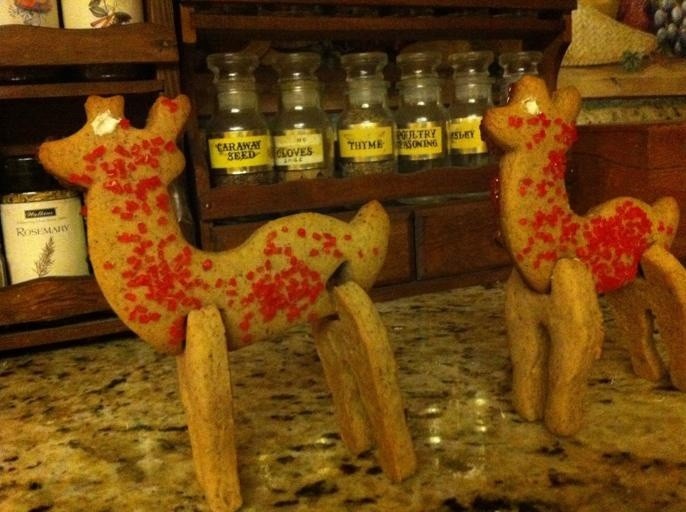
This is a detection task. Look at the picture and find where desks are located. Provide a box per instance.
[0,277,685,509]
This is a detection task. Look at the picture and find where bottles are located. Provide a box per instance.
[274,51,338,185]
[498,51,543,111]
[399,48,449,170]
[336,53,398,179]
[446,50,496,170]
[207,51,274,185]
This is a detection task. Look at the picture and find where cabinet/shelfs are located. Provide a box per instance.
[206,196,521,288]
[182,1,565,217]
[0,1,192,352]
